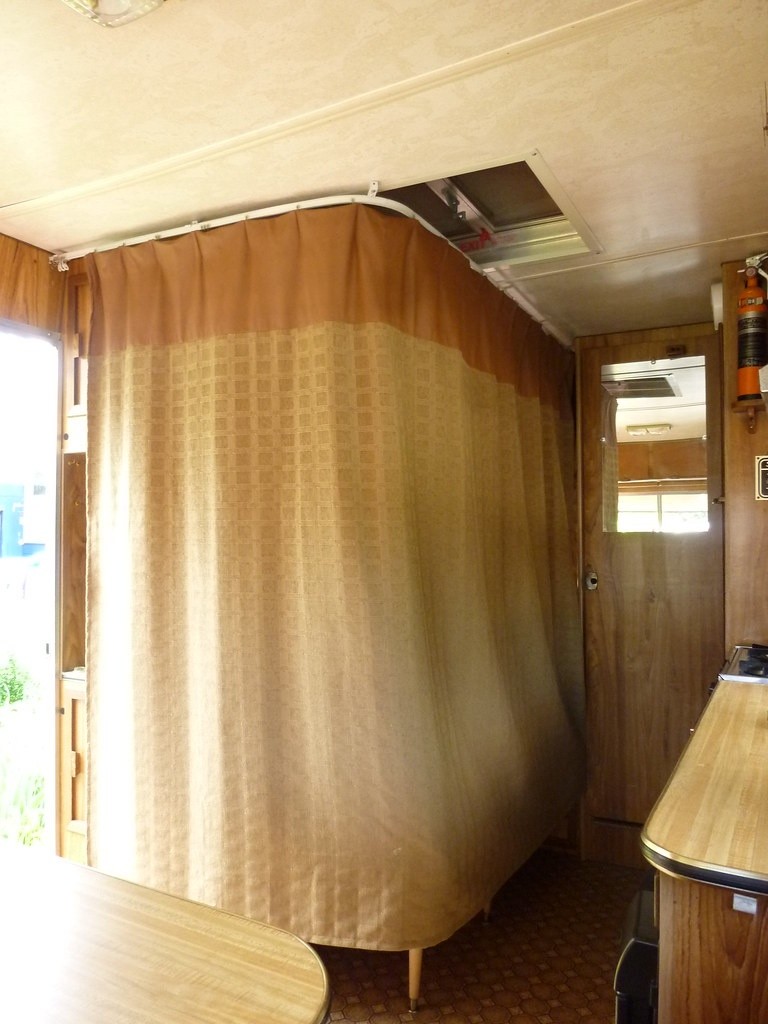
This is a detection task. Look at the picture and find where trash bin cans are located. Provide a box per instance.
[613,937,659,1024]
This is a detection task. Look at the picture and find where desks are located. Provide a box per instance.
[0,839,334,1024]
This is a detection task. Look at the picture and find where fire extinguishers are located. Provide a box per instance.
[738,251,768,401]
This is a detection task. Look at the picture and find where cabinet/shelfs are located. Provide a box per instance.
[641,678,768,1024]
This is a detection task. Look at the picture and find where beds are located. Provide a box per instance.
[43,196,594,1016]
